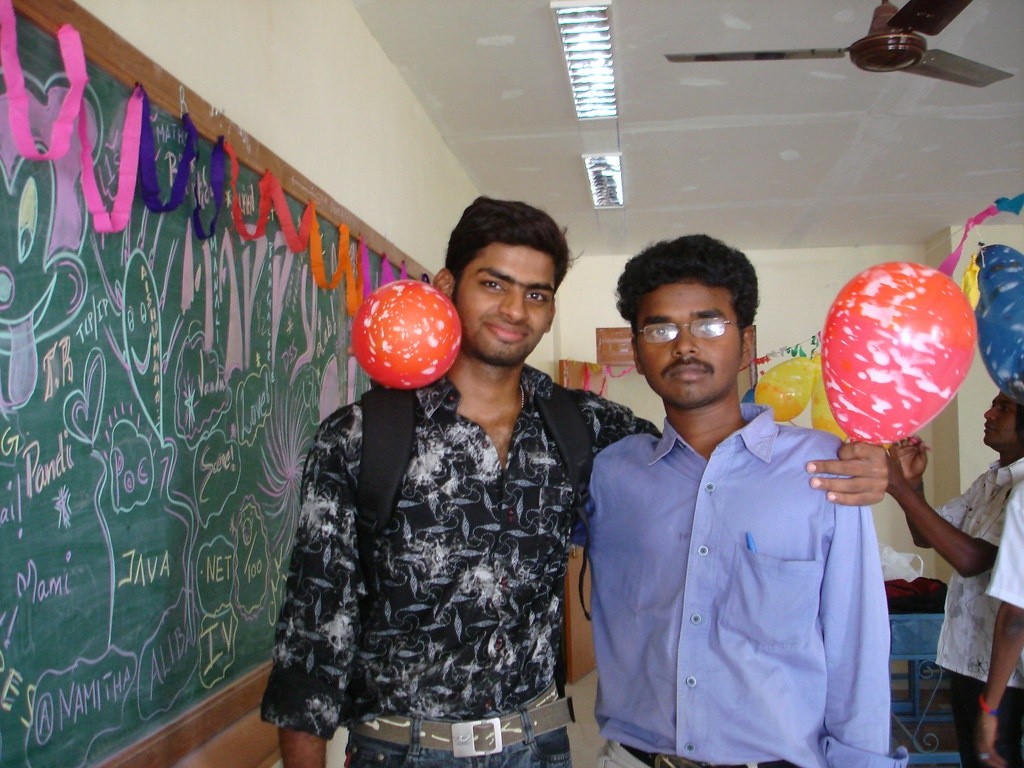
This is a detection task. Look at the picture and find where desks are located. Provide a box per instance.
[889,615,963,767]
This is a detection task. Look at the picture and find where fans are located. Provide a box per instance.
[663,0,1014,88]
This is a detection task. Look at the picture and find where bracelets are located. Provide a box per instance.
[978,691,1001,715]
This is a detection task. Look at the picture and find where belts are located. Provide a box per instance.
[352,679,575,757]
[618,744,800,768]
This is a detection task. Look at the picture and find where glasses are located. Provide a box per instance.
[638,317,740,344]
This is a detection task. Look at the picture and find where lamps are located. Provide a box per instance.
[550,0,617,119]
[581,152,632,210]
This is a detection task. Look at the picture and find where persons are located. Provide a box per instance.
[261,195,893,768]
[884,389,1024,768]
[583,234,892,768]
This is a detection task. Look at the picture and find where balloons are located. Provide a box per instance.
[820,261,978,449]
[752,352,850,443]
[965,240,1024,403]
[352,279,462,390]
